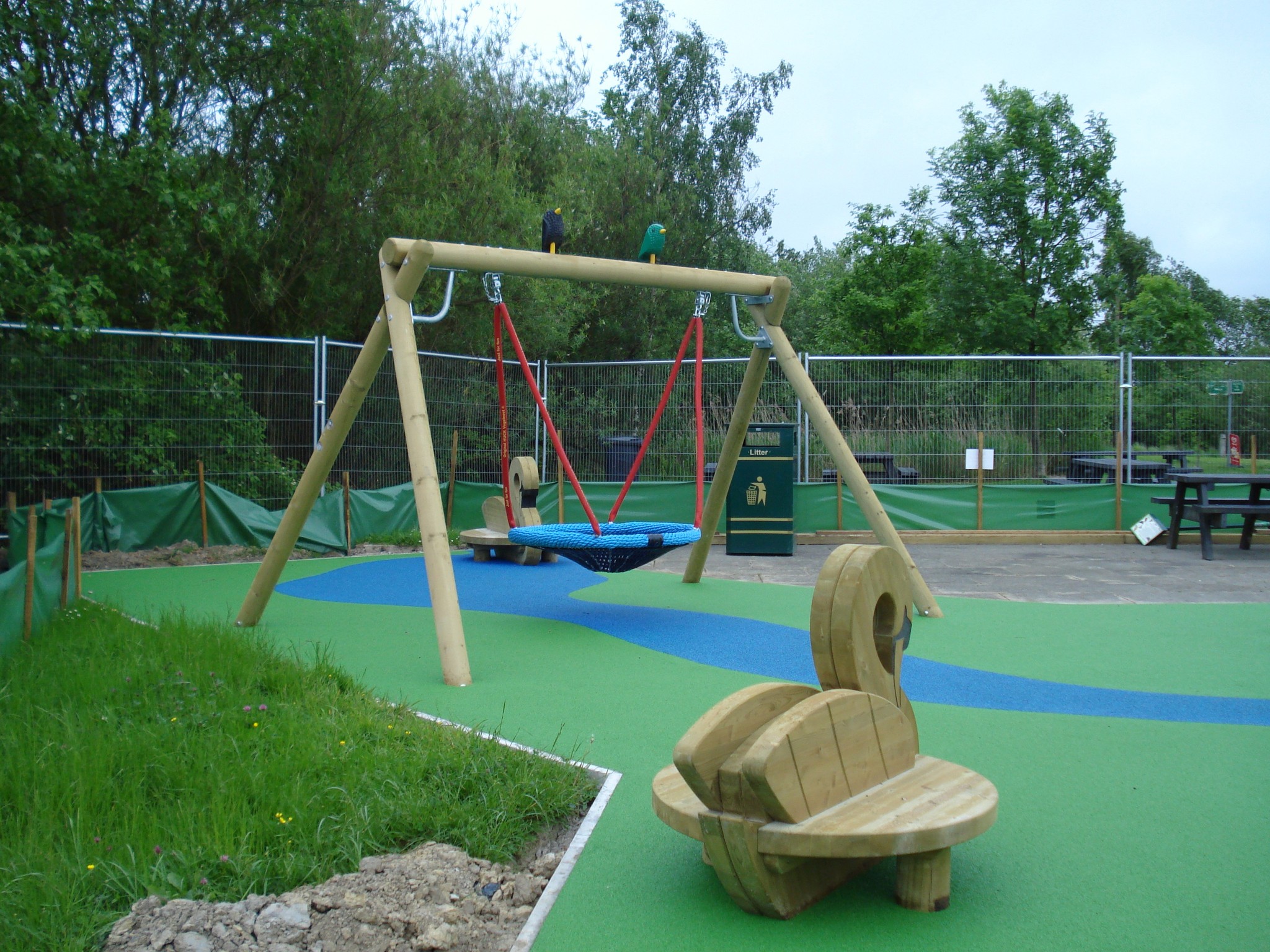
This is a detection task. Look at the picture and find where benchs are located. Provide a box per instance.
[822,470,837,475]
[1151,497,1270,506]
[1042,478,1077,485]
[1191,505,1270,515]
[896,467,921,477]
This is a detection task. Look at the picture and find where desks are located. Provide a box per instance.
[1164,474,1270,560]
[1071,458,1172,484]
[1061,450,1195,469]
[851,451,901,484]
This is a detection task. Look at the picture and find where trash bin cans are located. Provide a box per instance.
[603,437,643,482]
[724,423,799,556]
